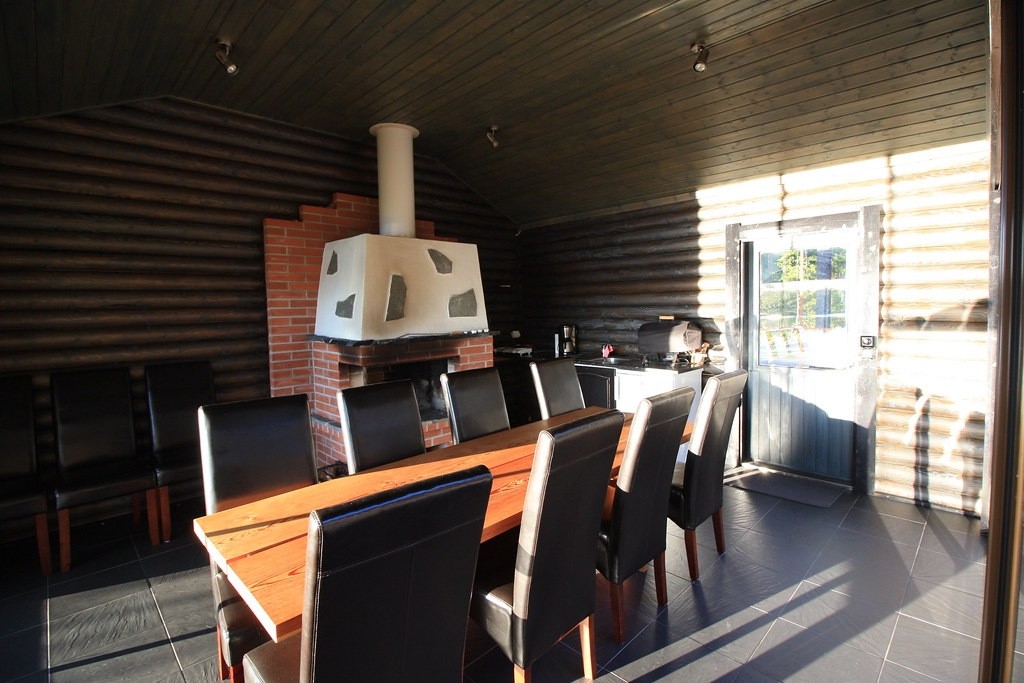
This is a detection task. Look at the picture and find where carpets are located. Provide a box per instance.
[731,470,847,509]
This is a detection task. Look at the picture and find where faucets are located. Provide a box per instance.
[599,343,617,362]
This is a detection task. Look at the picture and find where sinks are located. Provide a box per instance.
[580,357,630,364]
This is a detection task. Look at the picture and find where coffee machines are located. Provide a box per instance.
[560,325,576,356]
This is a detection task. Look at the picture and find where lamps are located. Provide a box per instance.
[214,38,240,76]
[691,42,709,73]
[486,126,499,149]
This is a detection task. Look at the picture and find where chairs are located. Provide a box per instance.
[52,367,159,573]
[242,465,492,683]
[440,366,510,446]
[145,357,218,543]
[529,358,586,420]
[472,409,625,683]
[198,393,317,683]
[596,386,697,644]
[337,379,427,478]
[0,376,50,576]
[638,369,748,581]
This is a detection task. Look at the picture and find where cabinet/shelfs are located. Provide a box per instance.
[616,371,704,463]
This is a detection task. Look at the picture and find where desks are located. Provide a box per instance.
[193,406,694,644]
